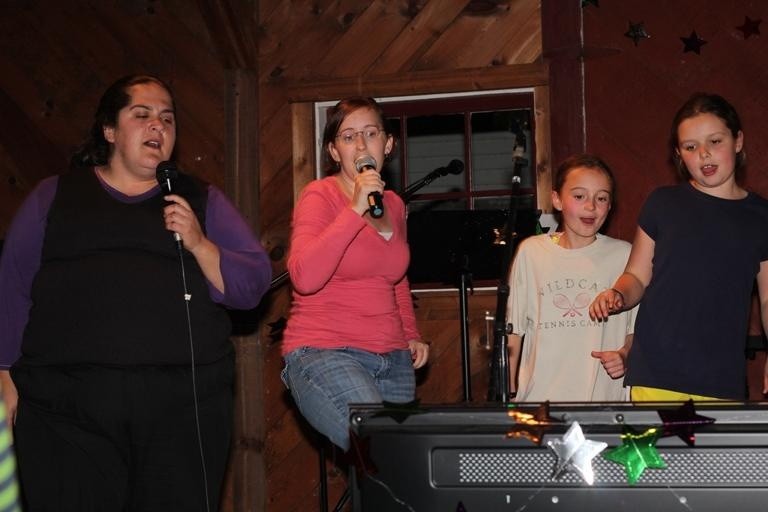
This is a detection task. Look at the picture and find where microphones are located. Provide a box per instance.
[426,159,464,180]
[156,160,182,250]
[355,157,384,217]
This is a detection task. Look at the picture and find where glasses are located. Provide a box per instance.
[335,127,384,142]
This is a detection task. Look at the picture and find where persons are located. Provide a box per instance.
[0,73,273,512]
[505,153,640,401]
[280,94,431,454]
[587,89,768,404]
